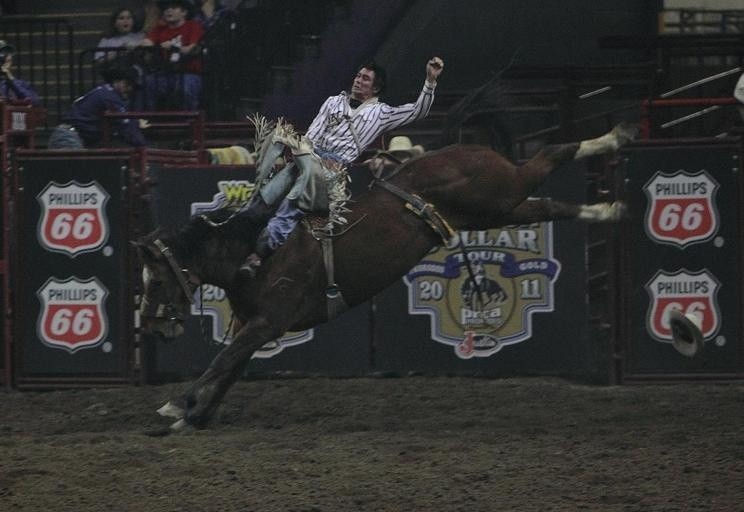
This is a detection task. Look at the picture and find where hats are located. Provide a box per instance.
[378,138,424,158]
[99,66,145,90]
[0,39,18,57]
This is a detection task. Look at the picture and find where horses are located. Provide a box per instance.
[126,77,642,439]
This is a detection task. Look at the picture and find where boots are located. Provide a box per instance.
[238,228,280,281]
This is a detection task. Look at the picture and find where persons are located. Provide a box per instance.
[0,1,223,150]
[231,55,444,282]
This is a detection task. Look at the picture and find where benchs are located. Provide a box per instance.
[0,1,238,150]
